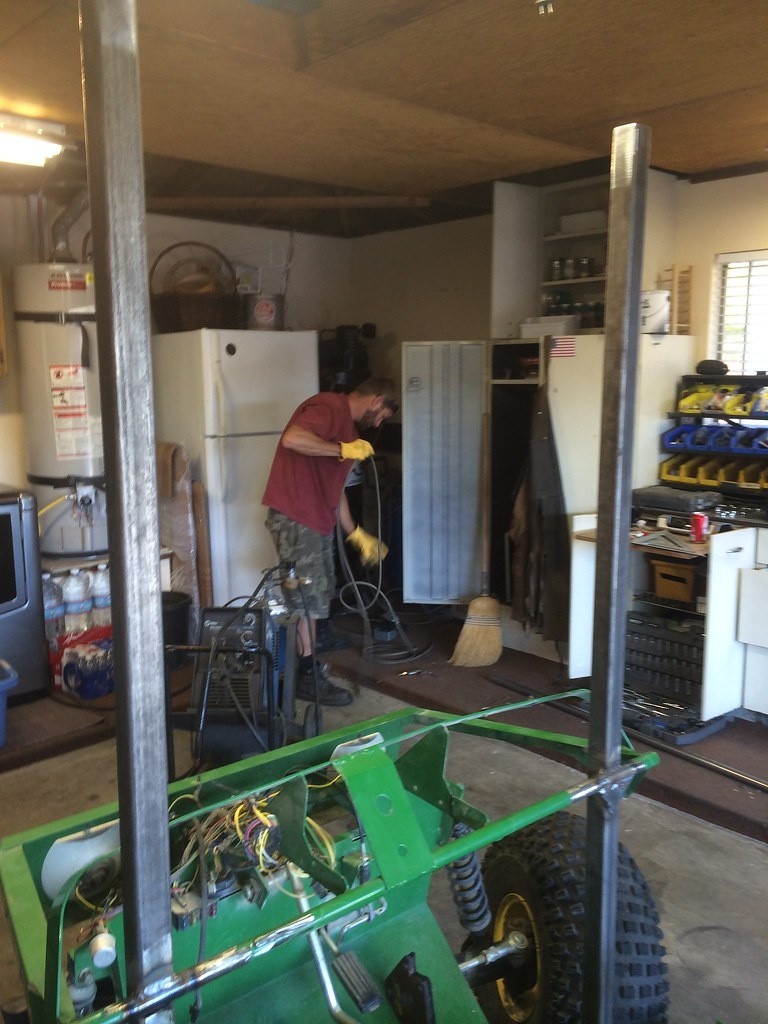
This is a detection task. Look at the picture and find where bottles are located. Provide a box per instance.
[40,564,112,643]
[550,258,603,329]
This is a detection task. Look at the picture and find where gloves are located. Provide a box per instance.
[345,523,389,568]
[338,439,375,461]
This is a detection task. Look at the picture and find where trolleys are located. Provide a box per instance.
[164,642,322,780]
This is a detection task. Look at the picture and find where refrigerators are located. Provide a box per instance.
[150,325,322,606]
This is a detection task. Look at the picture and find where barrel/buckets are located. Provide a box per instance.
[247,290,286,332]
[161,589,192,672]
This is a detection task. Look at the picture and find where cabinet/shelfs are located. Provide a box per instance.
[489,169,678,335]
[401,338,694,680]
[660,370,768,496]
[569,508,768,722]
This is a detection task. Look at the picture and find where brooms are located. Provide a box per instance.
[445,414,504,665]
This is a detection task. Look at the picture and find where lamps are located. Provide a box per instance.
[0,113,78,169]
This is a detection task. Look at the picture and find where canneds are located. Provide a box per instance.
[689,511,709,543]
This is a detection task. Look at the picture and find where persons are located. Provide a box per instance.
[262,377,399,706]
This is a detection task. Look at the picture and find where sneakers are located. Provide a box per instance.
[295,662,354,706]
[313,620,353,654]
